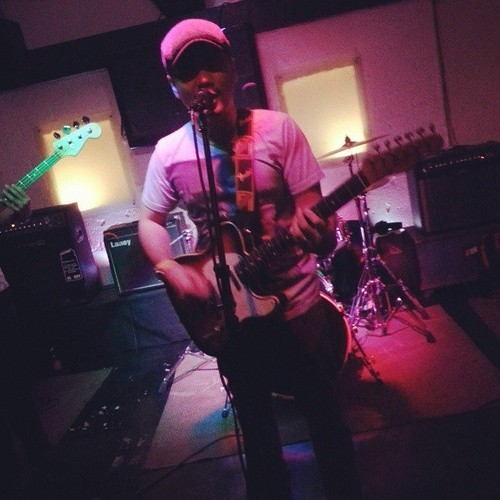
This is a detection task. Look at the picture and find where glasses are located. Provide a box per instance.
[177,52,224,83]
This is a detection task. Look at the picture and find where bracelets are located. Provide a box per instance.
[314,232,332,253]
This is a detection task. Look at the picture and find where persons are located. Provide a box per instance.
[138,18,365,500]
[0,183,52,468]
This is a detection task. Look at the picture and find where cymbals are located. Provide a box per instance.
[315,132,391,161]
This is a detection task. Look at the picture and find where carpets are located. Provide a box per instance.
[0,364,113,461]
[145,303,500,470]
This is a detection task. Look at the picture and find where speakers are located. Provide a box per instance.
[405,142,500,234]
[0,203,193,378]
[406,226,500,299]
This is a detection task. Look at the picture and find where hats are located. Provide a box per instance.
[161,19,233,72]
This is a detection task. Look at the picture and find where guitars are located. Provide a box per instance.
[0,115,102,213]
[162,123,445,356]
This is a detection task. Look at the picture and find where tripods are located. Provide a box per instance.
[344,156,436,344]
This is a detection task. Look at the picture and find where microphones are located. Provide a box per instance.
[194,87,216,109]
